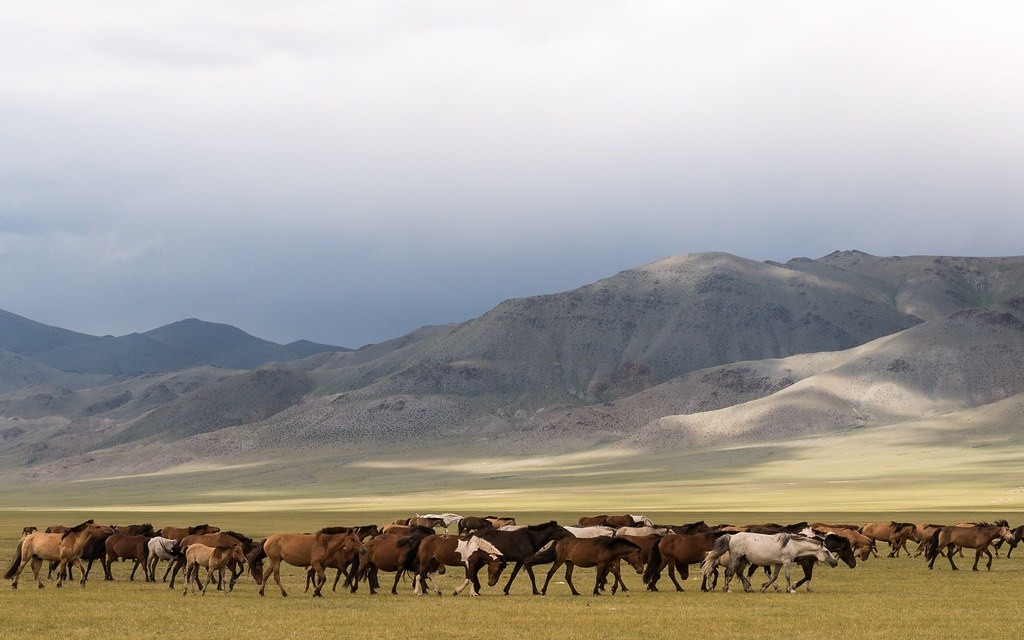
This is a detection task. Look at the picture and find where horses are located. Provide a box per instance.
[3,513,1024,597]
